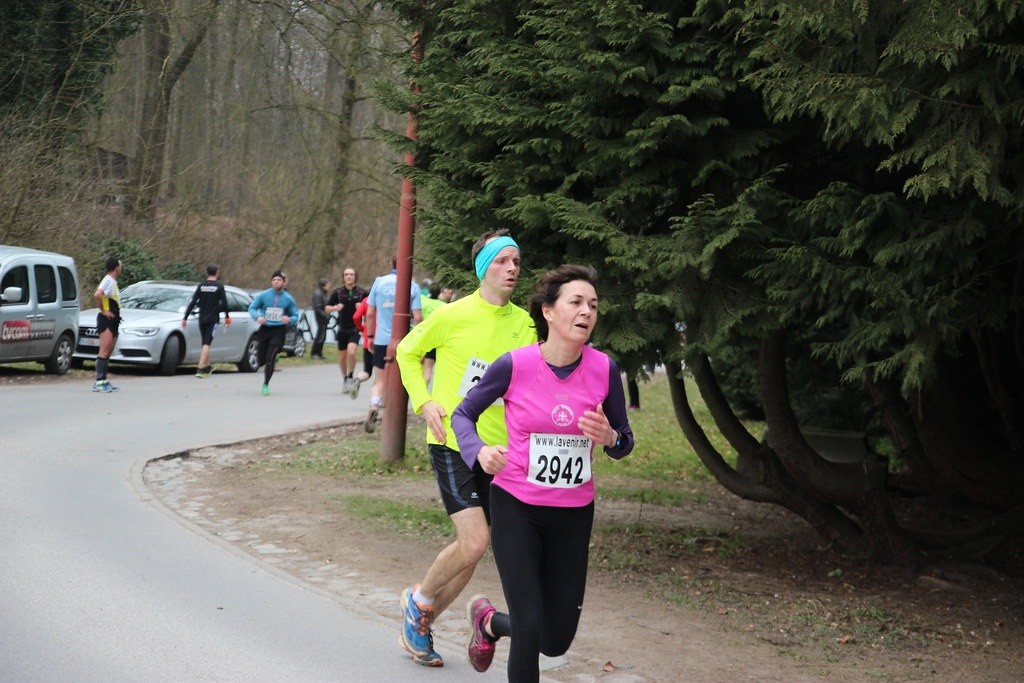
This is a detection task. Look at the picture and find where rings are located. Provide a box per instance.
[596,431,599,436]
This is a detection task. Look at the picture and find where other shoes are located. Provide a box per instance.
[261,384,269,395]
[348,377,360,400]
[309,354,321,360]
[274,355,279,362]
[363,406,379,434]
[341,377,354,393]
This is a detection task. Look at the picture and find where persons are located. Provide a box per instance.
[248,271,299,395]
[325,268,369,393]
[92,257,122,392]
[181,263,231,378]
[347,256,454,436]
[310,279,331,359]
[396,232,538,666]
[449,265,635,683]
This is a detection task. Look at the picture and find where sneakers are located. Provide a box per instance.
[465,594,500,673]
[195,364,216,378]
[92,380,113,393]
[399,581,444,667]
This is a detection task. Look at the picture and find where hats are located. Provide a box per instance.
[319,279,327,285]
[271,270,285,282]
[422,278,432,286]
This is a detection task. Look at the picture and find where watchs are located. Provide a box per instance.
[613,431,625,450]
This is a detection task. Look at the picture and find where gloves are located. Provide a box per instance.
[225,316,230,324]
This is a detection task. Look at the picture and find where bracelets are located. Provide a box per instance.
[609,429,618,447]
[367,335,374,338]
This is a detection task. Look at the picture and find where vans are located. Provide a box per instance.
[0,244,80,376]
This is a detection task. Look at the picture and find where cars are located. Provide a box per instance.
[280,324,307,358]
[70,279,264,376]
